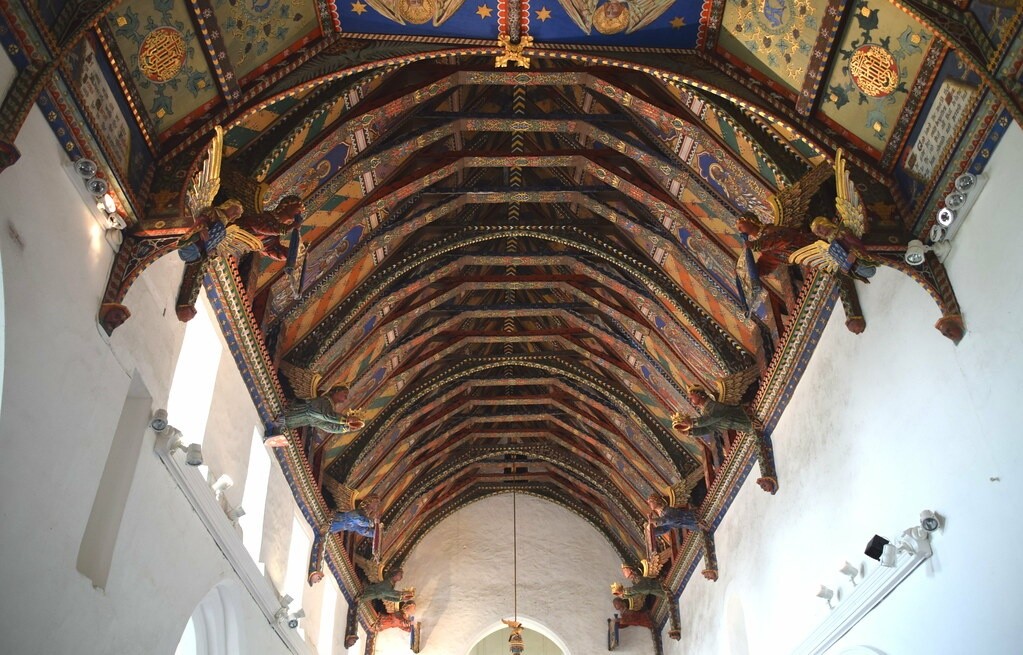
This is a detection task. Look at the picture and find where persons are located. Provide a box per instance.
[288,381,361,434]
[608,597,654,628]
[735,211,819,258]
[246,195,305,261]
[379,600,417,631]
[331,493,384,537]
[644,492,697,536]
[361,566,415,601]
[612,563,666,599]
[181,200,243,259]
[676,384,752,437]
[810,216,868,274]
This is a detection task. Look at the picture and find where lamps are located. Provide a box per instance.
[228,505,246,528]
[918,510,940,538]
[171,441,203,466]
[864,534,914,568]
[905,171,989,265]
[815,585,836,611]
[62,158,127,254]
[148,409,168,433]
[211,474,233,502]
[275,593,305,628]
[839,560,858,588]
